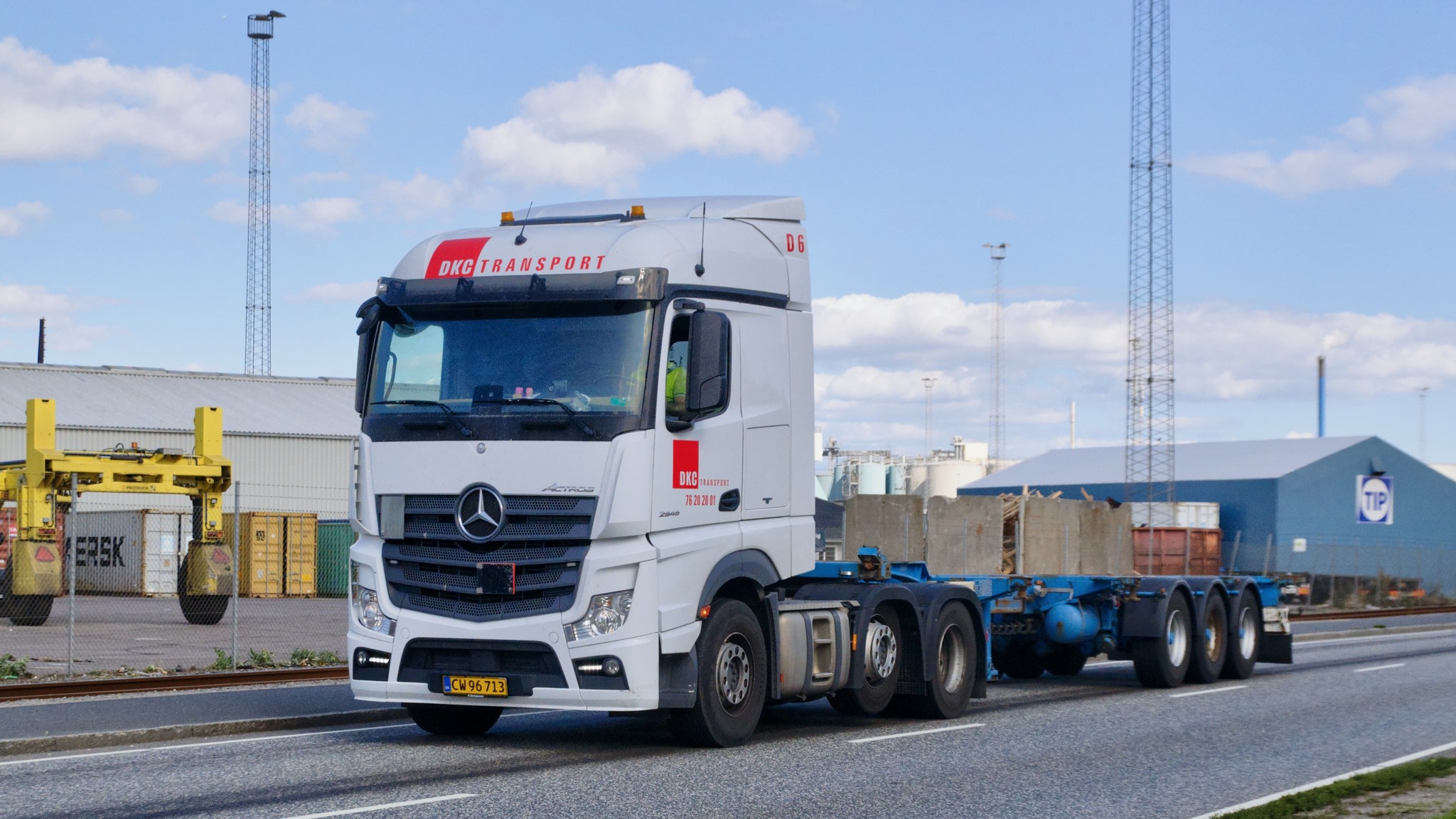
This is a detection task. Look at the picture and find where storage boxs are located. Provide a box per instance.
[1129,502,1222,576]
[63,509,195,598]
[317,519,359,598]
[0,507,65,595]
[221,512,318,599]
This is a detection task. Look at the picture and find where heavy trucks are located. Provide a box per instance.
[344,188,1293,749]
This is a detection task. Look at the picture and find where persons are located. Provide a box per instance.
[626,332,687,412]
